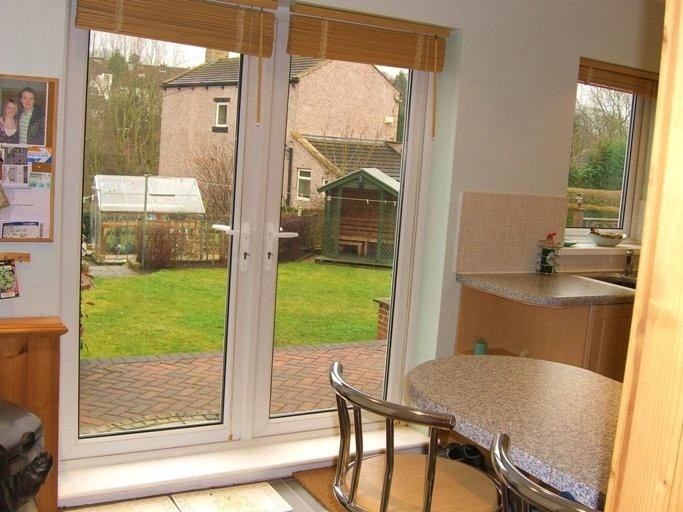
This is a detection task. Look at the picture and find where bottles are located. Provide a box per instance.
[538,233,556,275]
[624,249,632,277]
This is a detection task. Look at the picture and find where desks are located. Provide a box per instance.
[404,353,624,512]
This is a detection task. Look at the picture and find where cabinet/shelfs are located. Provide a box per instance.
[1,316,70,512]
[453,287,633,383]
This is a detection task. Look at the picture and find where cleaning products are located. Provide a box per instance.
[540,230,559,273]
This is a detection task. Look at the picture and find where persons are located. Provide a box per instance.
[15,86,45,146]
[0,98,19,145]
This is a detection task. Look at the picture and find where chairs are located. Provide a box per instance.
[486,429,602,512]
[324,360,504,512]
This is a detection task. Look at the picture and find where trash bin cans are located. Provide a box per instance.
[0,399,53,512]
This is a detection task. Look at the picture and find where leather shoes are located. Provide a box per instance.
[446,441,485,467]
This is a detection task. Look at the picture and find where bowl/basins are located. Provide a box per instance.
[585,232,628,247]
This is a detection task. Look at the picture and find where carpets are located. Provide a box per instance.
[291,467,349,512]
[169,481,293,512]
[64,495,179,512]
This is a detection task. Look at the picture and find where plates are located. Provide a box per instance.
[564,240,578,247]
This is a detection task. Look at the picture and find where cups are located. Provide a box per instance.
[474,342,488,355]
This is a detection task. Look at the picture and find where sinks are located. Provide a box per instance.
[567,271,637,292]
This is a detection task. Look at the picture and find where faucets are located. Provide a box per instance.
[622,249,635,275]
